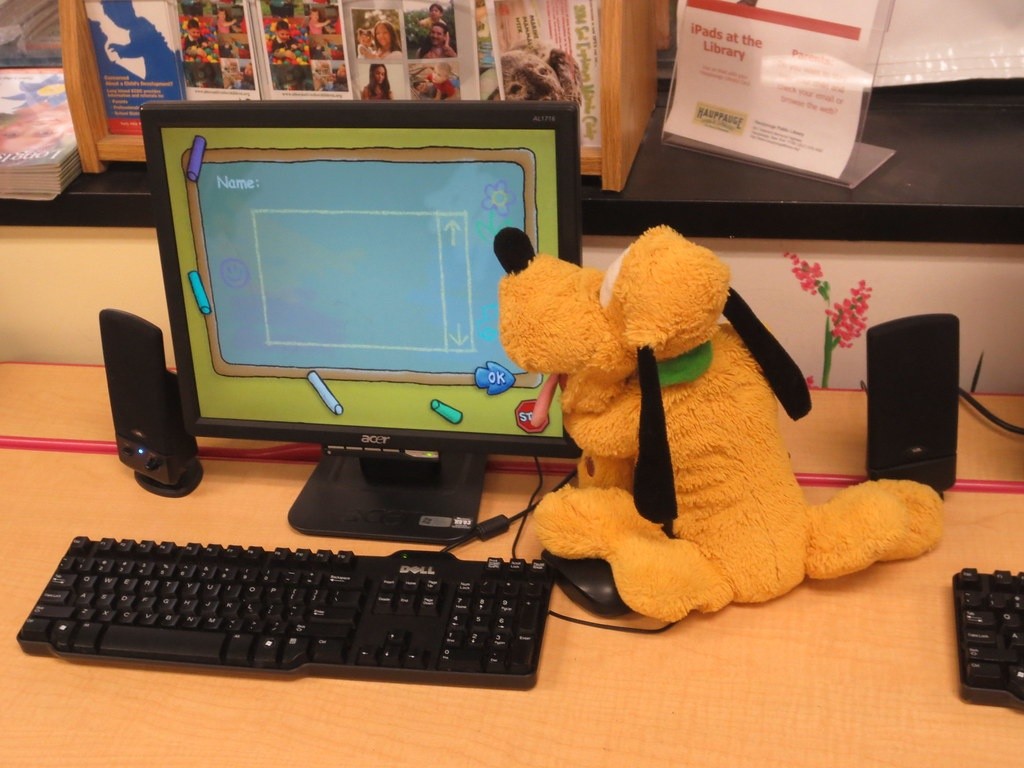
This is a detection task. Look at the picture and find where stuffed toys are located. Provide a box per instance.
[493,225,945,621]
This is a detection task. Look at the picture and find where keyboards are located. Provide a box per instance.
[951,568,1024,710]
[15,536,557,690]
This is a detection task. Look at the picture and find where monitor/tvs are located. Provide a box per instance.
[139,101,581,544]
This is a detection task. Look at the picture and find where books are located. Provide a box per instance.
[0,67,82,202]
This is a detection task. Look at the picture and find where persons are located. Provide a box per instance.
[176,0,349,98]
[0,103,72,154]
[356,2,461,101]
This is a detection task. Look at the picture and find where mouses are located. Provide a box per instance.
[540,548,633,619]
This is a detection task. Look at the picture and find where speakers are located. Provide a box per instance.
[99,309,206,497]
[865,313,960,497]
